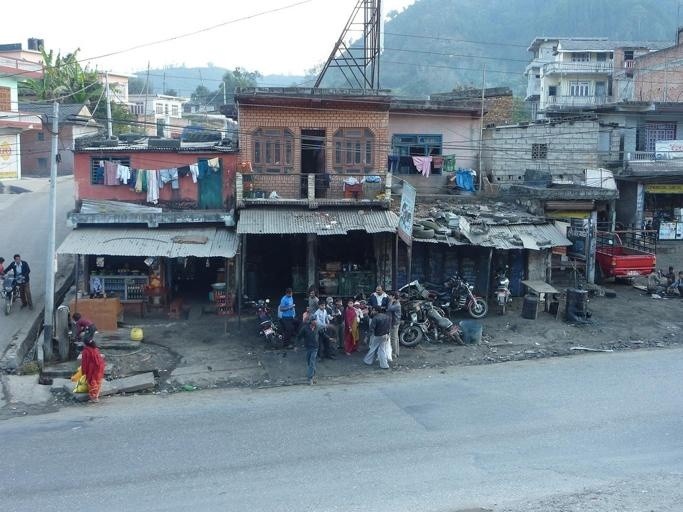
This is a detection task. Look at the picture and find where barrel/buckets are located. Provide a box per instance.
[565,289,589,322]
[130,327,143,341]
[522,293,538,319]
[459,321,482,345]
[73,342,85,359]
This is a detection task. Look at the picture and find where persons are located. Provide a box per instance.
[275,283,401,370]
[0,257,4,278]
[1,254,33,311]
[643,218,655,244]
[71,312,97,339]
[294,313,337,386]
[661,266,674,292]
[80,336,105,404]
[668,270,682,297]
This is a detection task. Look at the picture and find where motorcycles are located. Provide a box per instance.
[489,264,515,315]
[380,272,488,347]
[240,292,280,348]
[0,275,23,316]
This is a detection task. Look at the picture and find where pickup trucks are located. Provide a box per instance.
[561,221,658,283]
